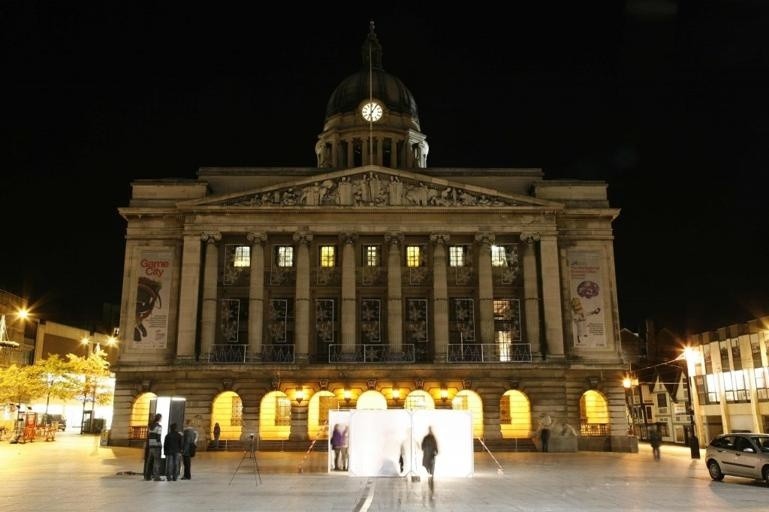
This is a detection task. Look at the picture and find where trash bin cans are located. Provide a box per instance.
[100,430,110,446]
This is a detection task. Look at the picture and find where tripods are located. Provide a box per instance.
[229,440,263,487]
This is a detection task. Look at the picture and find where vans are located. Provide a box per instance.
[47,414,67,430]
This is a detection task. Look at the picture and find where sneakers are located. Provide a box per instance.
[152,475,191,481]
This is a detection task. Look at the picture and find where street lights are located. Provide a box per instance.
[683,348,701,458]
[79,337,116,432]
[15,307,25,436]
[623,377,635,436]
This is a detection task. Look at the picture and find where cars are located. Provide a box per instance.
[705,433,769,484]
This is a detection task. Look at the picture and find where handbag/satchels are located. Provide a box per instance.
[188,442,197,458]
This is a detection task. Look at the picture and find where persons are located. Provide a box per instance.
[144,413,199,481]
[421,426,439,479]
[249,174,493,207]
[571,297,593,344]
[341,425,350,471]
[214,423,220,448]
[650,431,661,460]
[331,424,344,471]
[538,425,551,452]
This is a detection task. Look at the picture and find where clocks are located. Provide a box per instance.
[362,102,383,122]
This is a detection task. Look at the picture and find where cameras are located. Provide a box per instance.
[245,432,256,441]
